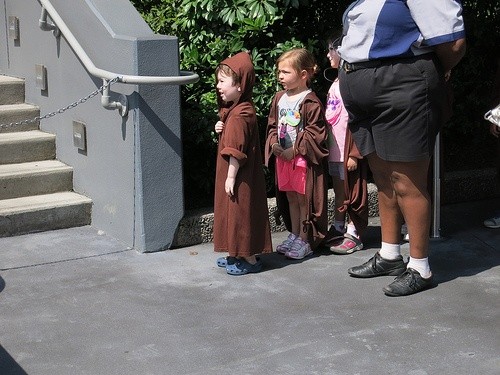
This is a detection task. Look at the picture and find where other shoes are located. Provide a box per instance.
[403,234,409,241]
[483,216,500,228]
[400,224,407,234]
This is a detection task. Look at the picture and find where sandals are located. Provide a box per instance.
[217,255,243,267]
[226,257,262,274]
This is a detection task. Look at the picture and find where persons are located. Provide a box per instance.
[265,48,329,259]
[323,36,368,254]
[340,1,466,296]
[213,52,273,274]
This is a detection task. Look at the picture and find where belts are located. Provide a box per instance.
[339,54,431,73]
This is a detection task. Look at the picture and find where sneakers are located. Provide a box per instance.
[330,233,363,254]
[285,238,313,260]
[276,233,299,254]
[382,268,438,296]
[325,225,346,242]
[348,253,406,278]
